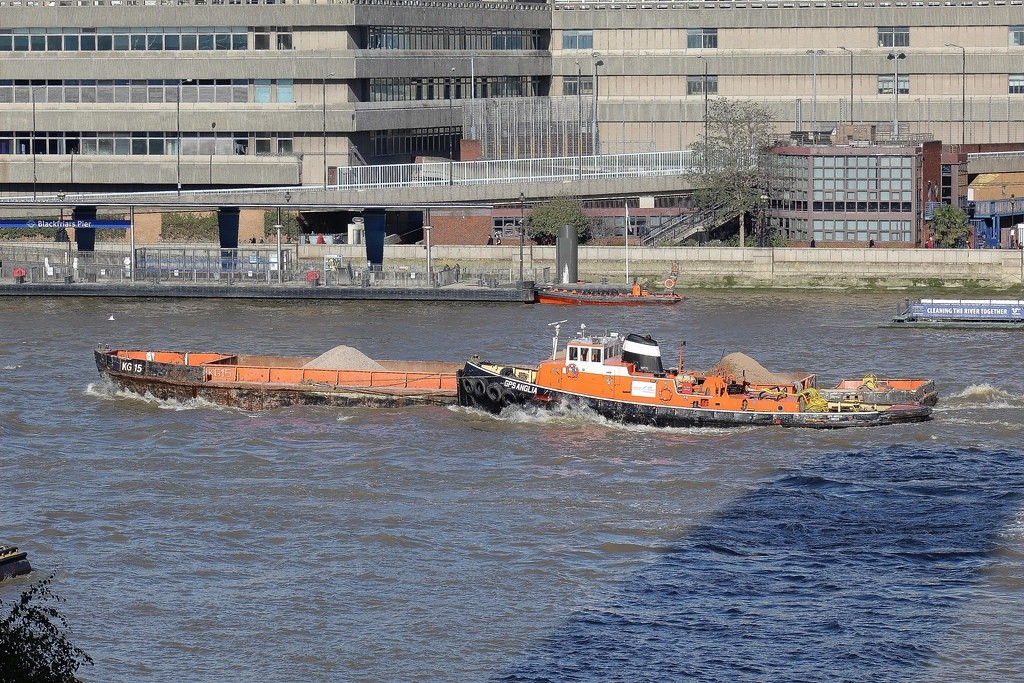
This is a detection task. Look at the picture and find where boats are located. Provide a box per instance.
[93,345,538,409]
[456,318,939,427]
[533,203,683,307]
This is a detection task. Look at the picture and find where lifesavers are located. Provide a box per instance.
[659,386,673,400]
[566,363,579,378]
[307,272,320,281]
[462,376,520,407]
[13,268,26,277]
[664,279,674,288]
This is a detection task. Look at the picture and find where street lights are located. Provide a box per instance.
[595,59,604,153]
[322,73,335,197]
[448,67,457,184]
[1008,193,1014,249]
[698,55,708,174]
[837,45,855,122]
[518,192,527,246]
[285,190,292,243]
[32,84,49,198]
[177,78,196,198]
[758,191,769,246]
[945,42,967,151]
[57,189,68,241]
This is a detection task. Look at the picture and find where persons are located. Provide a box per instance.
[810,236,815,248]
[925,238,932,248]
[250,234,264,243]
[487,234,501,245]
[869,237,875,248]
